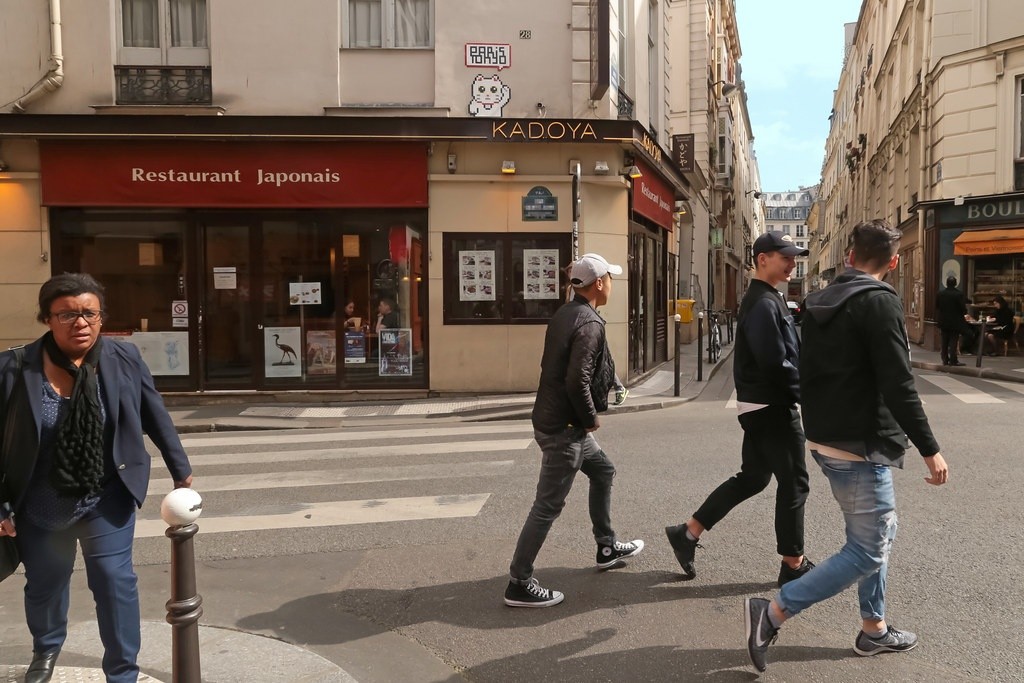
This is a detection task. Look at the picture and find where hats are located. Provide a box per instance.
[570,253,622,289]
[753,231,809,256]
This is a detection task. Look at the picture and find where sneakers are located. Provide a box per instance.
[744,598,780,673]
[504,577,564,608]
[778,555,816,588]
[596,540,644,569]
[665,522,706,578]
[853,624,918,656]
[613,387,629,405]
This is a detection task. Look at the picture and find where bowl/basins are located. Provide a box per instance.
[348,326,363,333]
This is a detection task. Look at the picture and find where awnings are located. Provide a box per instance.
[953,228,1024,255]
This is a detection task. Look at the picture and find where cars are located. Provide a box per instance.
[786,301,802,323]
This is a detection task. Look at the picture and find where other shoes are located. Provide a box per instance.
[990,351,1000,356]
[962,350,972,355]
[943,361,948,365]
[949,361,966,366]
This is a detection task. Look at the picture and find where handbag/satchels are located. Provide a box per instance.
[992,325,1009,332]
[0,512,20,583]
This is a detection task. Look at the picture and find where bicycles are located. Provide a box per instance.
[703,309,732,363]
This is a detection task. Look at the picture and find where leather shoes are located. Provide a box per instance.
[25,648,61,683]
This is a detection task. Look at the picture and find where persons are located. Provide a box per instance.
[343,297,355,329]
[934,276,979,366]
[375,297,398,333]
[666,230,816,588]
[0,272,193,683]
[502,252,645,609]
[743,219,948,675]
[985,295,1014,357]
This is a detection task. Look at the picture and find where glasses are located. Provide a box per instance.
[49,310,104,324]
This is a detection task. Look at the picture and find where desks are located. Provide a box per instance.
[966,319,998,356]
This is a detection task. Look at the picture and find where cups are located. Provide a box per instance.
[141,319,148,332]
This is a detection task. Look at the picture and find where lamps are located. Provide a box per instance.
[745,189,762,200]
[709,80,737,98]
[619,166,643,178]
[501,160,516,174]
[593,160,610,175]
[674,205,687,215]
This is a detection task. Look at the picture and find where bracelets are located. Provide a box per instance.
[347,319,351,326]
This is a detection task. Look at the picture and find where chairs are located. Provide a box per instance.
[997,315,1022,357]
